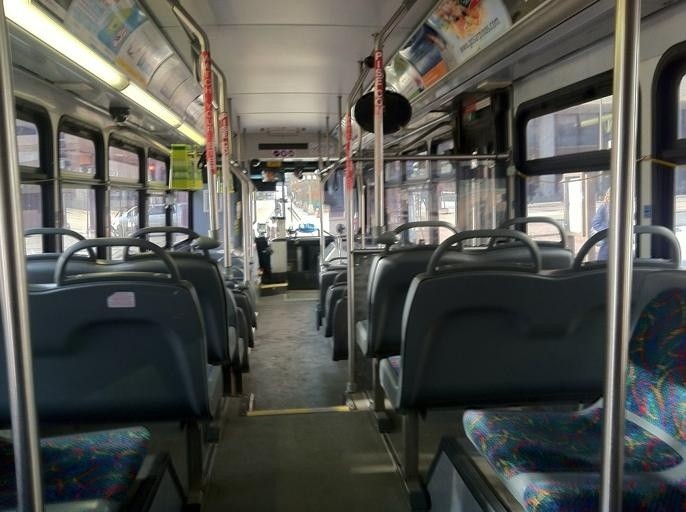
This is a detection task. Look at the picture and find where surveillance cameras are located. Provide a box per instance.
[109,108,130,122]
[249,159,261,168]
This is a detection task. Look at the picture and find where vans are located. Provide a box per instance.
[112,201,182,239]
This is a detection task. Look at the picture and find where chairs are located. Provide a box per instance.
[0,227,257,512]
[316,216,686,512]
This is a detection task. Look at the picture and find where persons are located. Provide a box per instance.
[591,186,611,261]
